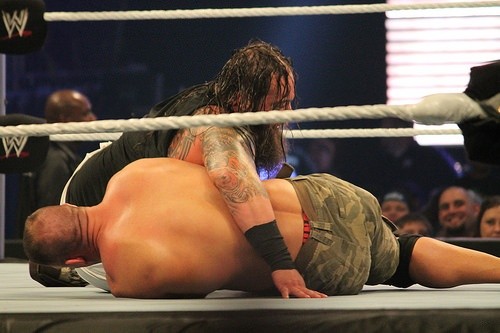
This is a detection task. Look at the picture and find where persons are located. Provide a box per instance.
[59,43,331,298]
[37,89,99,205]
[394,212,434,238]
[376,190,411,221]
[474,197,499,238]
[434,186,481,238]
[23,156,500,300]
